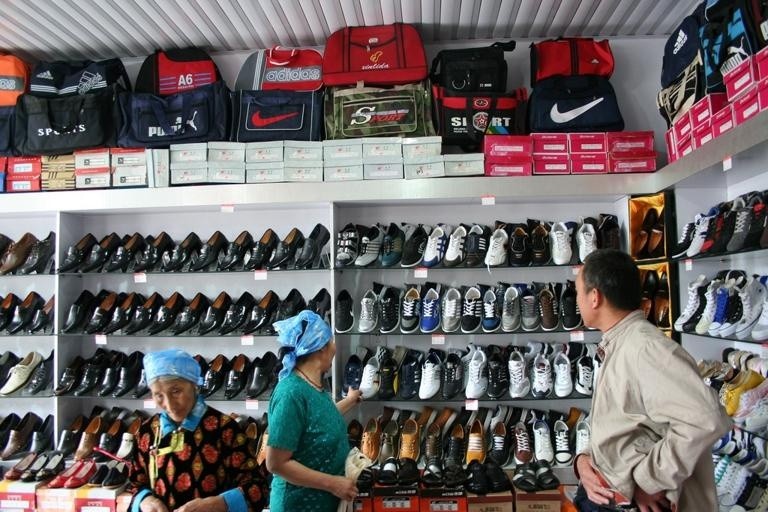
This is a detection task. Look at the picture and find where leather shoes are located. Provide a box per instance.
[267,289,308,334]
[1,295,17,331]
[121,233,133,243]
[29,350,56,394]
[28,414,54,452]
[53,355,86,396]
[191,230,229,270]
[640,269,658,323]
[117,291,128,304]
[128,410,149,423]
[7,292,46,334]
[93,418,126,461]
[102,292,144,335]
[121,292,165,335]
[60,289,94,333]
[72,348,113,397]
[134,232,177,270]
[295,223,331,267]
[246,349,278,398]
[132,369,150,400]
[90,405,103,419]
[2,413,42,460]
[245,229,282,270]
[190,352,208,378]
[76,231,122,270]
[242,290,281,335]
[0,352,20,386]
[224,353,252,400]
[57,233,99,270]
[0,411,20,449]
[219,290,258,335]
[146,291,187,336]
[75,417,108,459]
[96,351,128,397]
[164,232,201,271]
[197,291,234,334]
[655,270,669,326]
[117,416,143,459]
[111,406,129,421]
[199,354,231,400]
[85,291,119,333]
[146,234,158,242]
[228,353,238,366]
[266,227,305,268]
[310,289,331,319]
[84,289,109,324]
[111,349,146,399]
[0,231,11,254]
[139,291,148,301]
[55,415,89,457]
[251,354,261,371]
[30,296,55,334]
[102,233,147,273]
[174,292,212,335]
[20,230,58,273]
[220,232,255,268]
[0,234,39,275]
[0,353,42,395]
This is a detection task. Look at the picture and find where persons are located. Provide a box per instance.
[572,248,736,512]
[264,310,363,512]
[126,348,273,512]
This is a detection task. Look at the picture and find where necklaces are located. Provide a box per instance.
[294,365,324,389]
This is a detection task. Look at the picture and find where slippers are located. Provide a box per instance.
[531,458,561,490]
[511,462,539,492]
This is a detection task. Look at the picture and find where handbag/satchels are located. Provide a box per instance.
[16,94,117,150]
[127,44,231,95]
[321,21,430,84]
[660,15,702,87]
[652,56,707,132]
[324,81,434,140]
[526,25,616,78]
[0,50,30,107]
[233,89,324,141]
[14,56,131,95]
[432,35,518,89]
[700,4,759,90]
[232,47,324,90]
[119,83,231,147]
[526,75,625,134]
[434,86,525,148]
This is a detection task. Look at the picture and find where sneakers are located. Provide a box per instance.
[47,459,84,489]
[396,409,418,428]
[445,424,468,460]
[732,378,768,423]
[561,285,583,330]
[443,353,466,400]
[602,215,622,252]
[548,341,570,365]
[578,221,597,263]
[503,287,520,332]
[458,342,483,394]
[445,455,466,486]
[714,449,754,484]
[376,357,400,399]
[466,418,487,464]
[392,344,409,369]
[400,358,421,398]
[716,457,756,496]
[567,342,587,364]
[585,340,600,364]
[406,283,418,291]
[487,342,506,362]
[465,348,490,399]
[380,407,402,425]
[443,227,470,267]
[491,422,512,467]
[378,457,399,486]
[429,349,446,362]
[534,420,555,466]
[439,284,461,334]
[462,286,482,333]
[373,281,388,296]
[485,458,509,492]
[477,407,495,433]
[500,279,511,291]
[533,354,553,399]
[712,440,740,468]
[354,225,386,266]
[398,419,422,462]
[718,276,748,338]
[458,407,479,432]
[354,344,372,362]
[488,404,515,434]
[548,410,562,433]
[554,351,573,398]
[358,288,379,332]
[356,462,378,490]
[695,276,726,335]
[576,422,590,456]
[718,458,768,512]
[727,370,763,417]
[484,228,510,267]
[746,393,768,431]
[4,452,37,480]
[555,280,567,318]
[380,420,401,460]
[20,453,49,482]
[486,352,509,398]
[401,224,429,267]
[670,221,696,258]
[734,276,766,339]
[409,350,425,359]
[417,352,445,401]
[540,290,558,332]
[505,343,527,357]
[380,285,403,333]
[466,225,488,264]
[381,223,405,266]
[727,194,766,252]
[432,406,458,440]
[374,345,391,366]
[683,274,721,331]
[334,224,361,268]
[87,459,118,487]
[647,205,667,255]
[535,282,548,296]
[515,422,533,463]
[673,272,708,331]
[531,224,550,268]
[560,407,588,444]
[333,288,354,333]
[710,193,750,254]
[713,368,740,391]
[687,205,722,258]
[730,472,768,511]
[522,288,539,331]
[550,222,573,266]
[338,354,364,399]
[425,282,441,291]
[35,453,65,481]
[528,409,546,427]
[632,207,657,258]
[103,462,127,486]
[397,456,420,485]
[360,417,384,465]
[510,407,529,430]
[446,349,467,358]
[510,224,529,267]
[350,420,362,439]
[396,286,420,333]
[706,277,739,337]
[507,350,532,399]
[477,283,494,296]
[523,341,550,372]
[357,358,382,399]
[574,355,595,397]
[480,289,501,334]
[422,225,447,268]
[515,283,533,292]
[64,459,97,489]
[418,286,438,332]
[417,406,438,439]
[698,202,733,252]
[719,370,746,405]
[552,420,573,467]
[424,422,442,461]
[752,293,768,341]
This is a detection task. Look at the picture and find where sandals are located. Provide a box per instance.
[421,456,443,486]
[463,460,489,495]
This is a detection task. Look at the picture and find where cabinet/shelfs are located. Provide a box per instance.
[670,106,768,511]
[334,164,673,475]
[0,190,60,453]
[59,181,336,472]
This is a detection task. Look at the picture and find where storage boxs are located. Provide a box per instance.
[9,157,41,192]
[145,148,170,189]
[723,46,768,130]
[403,136,445,181]
[443,152,486,177]
[363,137,403,180]
[209,142,245,185]
[484,135,535,177]
[535,134,571,173]
[40,172,76,190]
[322,137,363,157]
[41,155,75,173]
[245,140,284,164]
[247,161,285,183]
[0,156,6,193]
[112,148,147,188]
[570,133,611,173]
[665,94,733,165]
[171,142,207,184]
[608,132,659,174]
[286,142,323,184]
[325,159,363,180]
[350,485,563,510]
[1,476,130,512]
[74,147,109,169]
